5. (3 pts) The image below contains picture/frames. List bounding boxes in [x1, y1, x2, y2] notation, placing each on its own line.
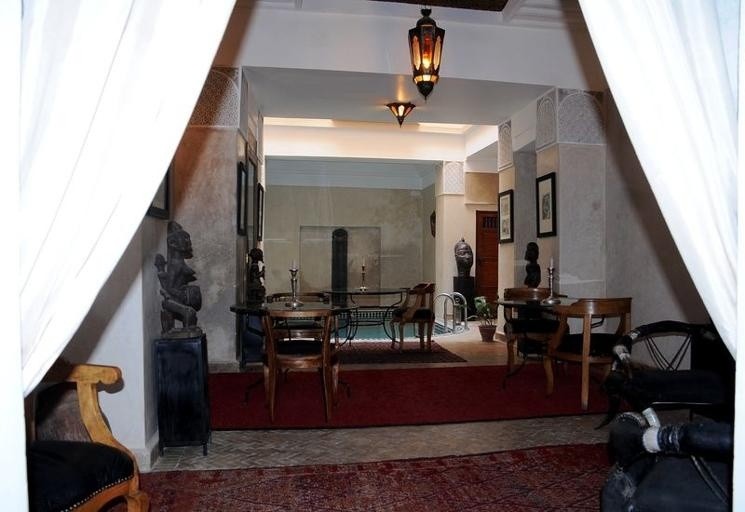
[149, 172, 172, 220]
[536, 172, 556, 236]
[257, 183, 263, 240]
[498, 189, 514, 242]
[238, 161, 246, 235]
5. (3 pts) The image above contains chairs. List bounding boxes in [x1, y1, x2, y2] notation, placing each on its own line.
[260, 284, 435, 423]
[612, 320, 731, 423]
[503, 288, 633, 410]
[24, 364, 150, 512]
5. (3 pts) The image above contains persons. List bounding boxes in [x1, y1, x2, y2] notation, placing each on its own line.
[454, 238, 474, 279]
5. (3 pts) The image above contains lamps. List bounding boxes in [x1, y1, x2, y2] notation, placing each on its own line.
[386, 105, 414, 128]
[407, 7, 445, 105]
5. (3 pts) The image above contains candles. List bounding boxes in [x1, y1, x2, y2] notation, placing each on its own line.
[549, 253, 554, 270]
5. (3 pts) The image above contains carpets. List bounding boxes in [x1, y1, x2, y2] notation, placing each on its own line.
[101, 442, 611, 512]
[327, 340, 468, 364]
[208, 362, 637, 431]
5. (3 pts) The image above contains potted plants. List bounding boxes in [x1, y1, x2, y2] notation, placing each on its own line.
[459, 300, 498, 341]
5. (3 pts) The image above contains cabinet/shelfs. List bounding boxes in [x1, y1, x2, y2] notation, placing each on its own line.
[239, 315, 267, 369]
